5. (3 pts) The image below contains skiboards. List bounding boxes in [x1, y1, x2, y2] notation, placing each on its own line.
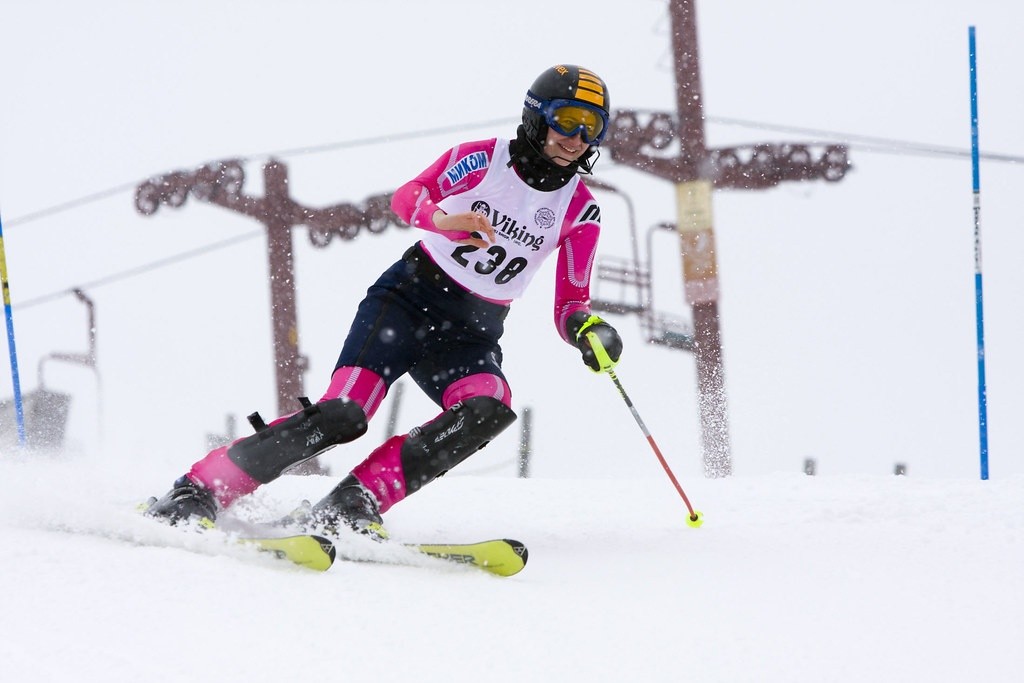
[26, 500, 529, 579]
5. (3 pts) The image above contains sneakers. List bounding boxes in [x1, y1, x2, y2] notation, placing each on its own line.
[313, 474, 390, 540]
[144, 475, 219, 532]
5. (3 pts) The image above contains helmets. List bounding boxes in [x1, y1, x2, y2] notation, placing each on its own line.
[523, 65, 610, 145]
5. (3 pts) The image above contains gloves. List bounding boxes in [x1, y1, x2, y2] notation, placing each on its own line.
[567, 313, 621, 371]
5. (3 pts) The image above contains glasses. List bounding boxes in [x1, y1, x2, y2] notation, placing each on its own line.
[525, 90, 609, 146]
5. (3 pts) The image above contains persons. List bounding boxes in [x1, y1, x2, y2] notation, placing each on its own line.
[151, 65, 622, 540]
